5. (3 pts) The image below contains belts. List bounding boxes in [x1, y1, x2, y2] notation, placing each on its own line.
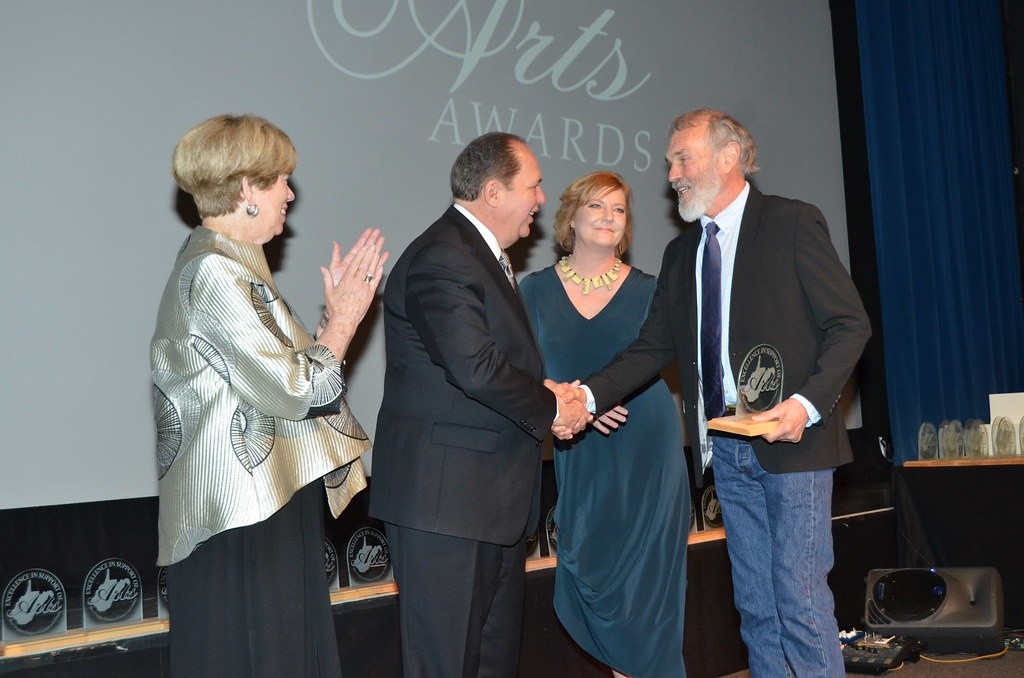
[723, 407, 736, 419]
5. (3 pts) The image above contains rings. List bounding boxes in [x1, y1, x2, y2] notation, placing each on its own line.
[365, 276, 373, 283]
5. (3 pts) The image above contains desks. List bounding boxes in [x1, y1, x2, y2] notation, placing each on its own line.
[893, 457, 1024, 633]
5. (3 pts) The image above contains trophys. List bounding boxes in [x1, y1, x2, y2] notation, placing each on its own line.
[707, 344, 784, 437]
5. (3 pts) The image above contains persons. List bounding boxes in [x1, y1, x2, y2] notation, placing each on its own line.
[368, 132, 591, 678]
[520, 172, 687, 678]
[559, 108, 874, 678]
[151, 114, 389, 678]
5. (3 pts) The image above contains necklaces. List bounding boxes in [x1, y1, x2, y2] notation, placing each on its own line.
[559, 253, 622, 295]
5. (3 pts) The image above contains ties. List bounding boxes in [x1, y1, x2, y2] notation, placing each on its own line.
[500, 249, 517, 296]
[701, 222, 725, 418]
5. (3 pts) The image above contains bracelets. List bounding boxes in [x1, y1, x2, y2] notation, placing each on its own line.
[320, 305, 329, 328]
